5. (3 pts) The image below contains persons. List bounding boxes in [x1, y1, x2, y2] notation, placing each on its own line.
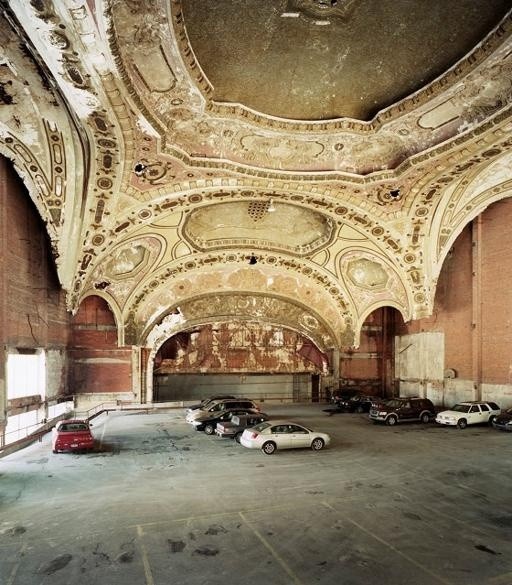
[325, 387, 331, 402]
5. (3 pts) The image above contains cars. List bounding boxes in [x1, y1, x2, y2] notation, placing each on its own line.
[239, 419, 330, 454]
[52, 420, 95, 453]
[331, 388, 384, 413]
[435, 401, 502, 430]
[186, 395, 270, 444]
[369, 397, 436, 425]
[492, 407, 512, 431]
